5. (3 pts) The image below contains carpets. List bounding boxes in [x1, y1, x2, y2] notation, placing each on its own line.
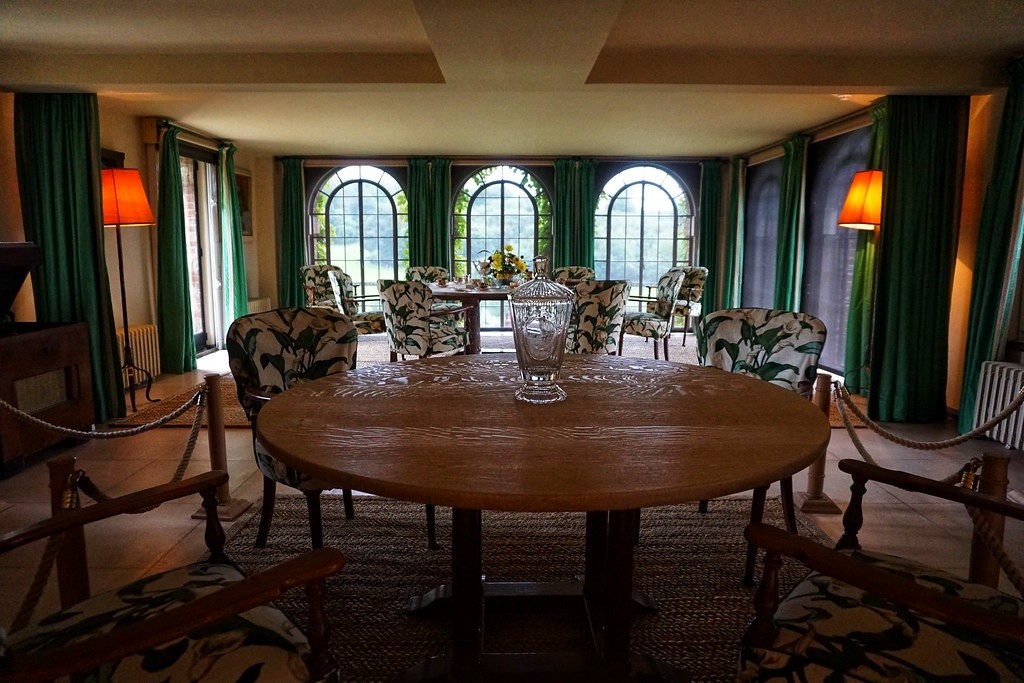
[812, 393, 868, 429]
[107, 369, 252, 429]
[195, 491, 837, 682]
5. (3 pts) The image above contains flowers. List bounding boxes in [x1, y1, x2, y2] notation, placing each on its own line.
[477, 245, 527, 284]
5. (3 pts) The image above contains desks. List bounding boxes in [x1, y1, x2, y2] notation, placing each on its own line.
[255, 353, 831, 682]
[427, 279, 514, 355]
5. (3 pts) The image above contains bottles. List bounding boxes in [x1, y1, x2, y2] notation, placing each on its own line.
[506, 252, 576, 407]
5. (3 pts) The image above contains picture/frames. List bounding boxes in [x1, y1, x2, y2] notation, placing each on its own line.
[233, 167, 255, 242]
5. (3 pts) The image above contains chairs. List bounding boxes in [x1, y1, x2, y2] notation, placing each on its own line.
[0, 468, 345, 683]
[696, 307, 827, 587]
[645, 267, 709, 347]
[619, 271, 686, 362]
[300, 264, 474, 362]
[737, 458, 1024, 683]
[226, 306, 441, 592]
[551, 266, 632, 356]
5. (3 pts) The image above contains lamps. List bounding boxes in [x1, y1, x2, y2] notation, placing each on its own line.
[100, 168, 161, 414]
[838, 170, 883, 396]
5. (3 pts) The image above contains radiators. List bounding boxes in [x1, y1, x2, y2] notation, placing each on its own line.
[115, 325, 161, 388]
[248, 297, 272, 314]
[971, 362, 1024, 450]
